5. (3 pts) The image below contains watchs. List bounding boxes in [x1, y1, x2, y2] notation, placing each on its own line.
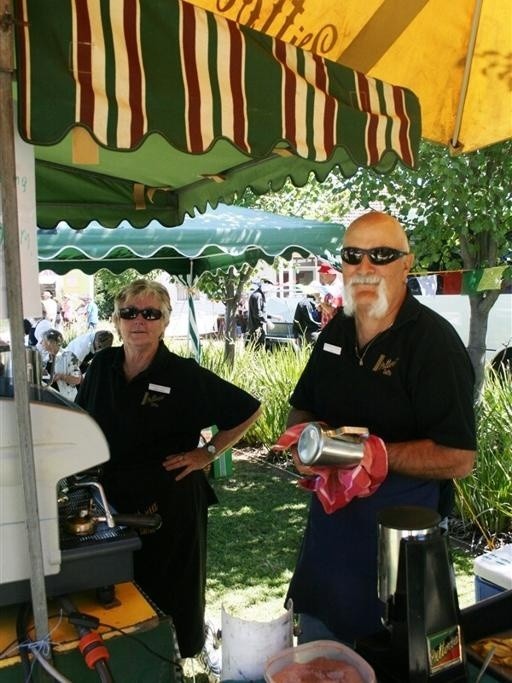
[202, 440, 220, 461]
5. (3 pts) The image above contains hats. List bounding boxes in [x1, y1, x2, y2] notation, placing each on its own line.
[317, 263, 338, 274]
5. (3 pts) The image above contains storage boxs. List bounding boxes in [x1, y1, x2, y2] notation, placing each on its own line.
[473, 542, 512, 603]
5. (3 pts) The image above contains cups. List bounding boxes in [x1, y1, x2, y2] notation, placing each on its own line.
[298, 422, 369, 471]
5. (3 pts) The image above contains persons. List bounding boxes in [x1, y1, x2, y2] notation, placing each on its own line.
[74, 277, 264, 668]
[235, 280, 342, 353]
[286, 210, 478, 652]
[1, 290, 116, 404]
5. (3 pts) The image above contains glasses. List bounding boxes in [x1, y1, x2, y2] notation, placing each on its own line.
[119, 307, 162, 320]
[341, 247, 408, 265]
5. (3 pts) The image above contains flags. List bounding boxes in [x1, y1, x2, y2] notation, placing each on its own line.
[411, 266, 509, 296]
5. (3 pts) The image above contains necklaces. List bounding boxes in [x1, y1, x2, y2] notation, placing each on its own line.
[354, 321, 392, 367]
